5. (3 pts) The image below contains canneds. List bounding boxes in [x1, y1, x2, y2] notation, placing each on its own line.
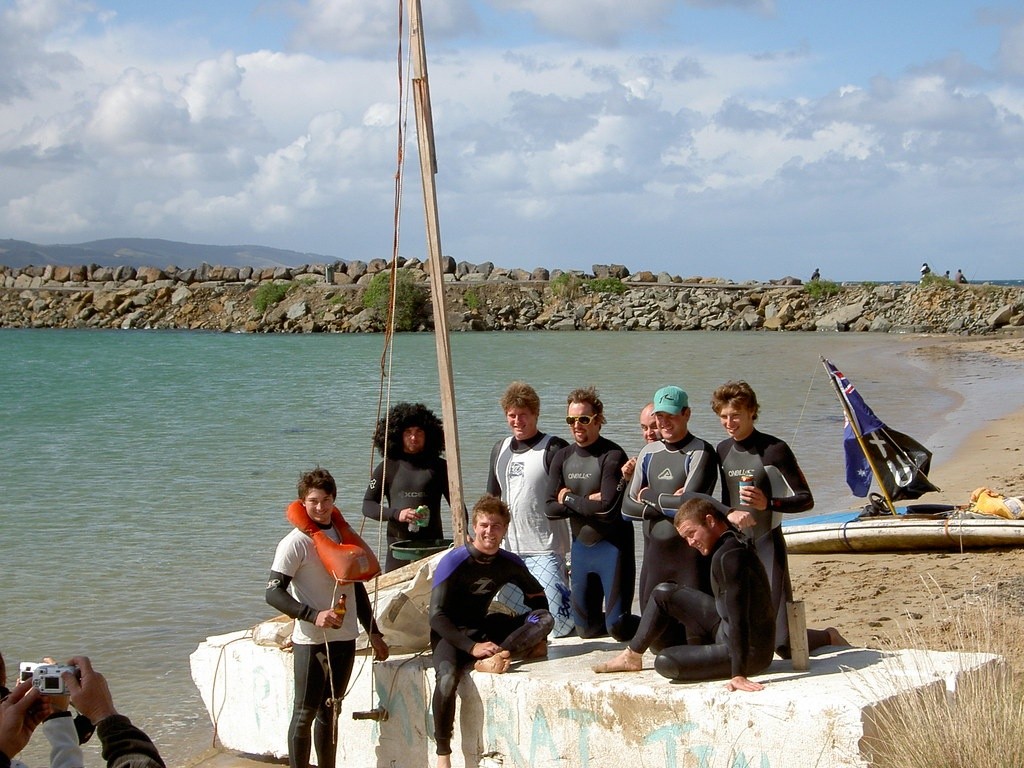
[739, 475, 755, 506]
[408, 505, 430, 532]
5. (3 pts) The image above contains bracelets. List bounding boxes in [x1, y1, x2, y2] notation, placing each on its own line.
[472, 643, 479, 652]
[90, 715, 110, 727]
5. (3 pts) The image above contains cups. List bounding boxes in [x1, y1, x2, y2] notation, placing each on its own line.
[739, 475, 754, 506]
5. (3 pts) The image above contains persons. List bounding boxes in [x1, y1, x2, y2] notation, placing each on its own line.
[0, 651, 166, 768]
[710, 380, 853, 660]
[590, 497, 774, 693]
[620, 386, 720, 656]
[921, 263, 932, 278]
[956, 269, 969, 284]
[363, 404, 474, 573]
[543, 385, 642, 644]
[941, 271, 950, 279]
[486, 381, 571, 638]
[811, 268, 820, 281]
[620, 402, 664, 614]
[428, 494, 554, 768]
[265, 472, 388, 768]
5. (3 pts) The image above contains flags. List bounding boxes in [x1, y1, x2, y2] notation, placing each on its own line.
[827, 359, 884, 501]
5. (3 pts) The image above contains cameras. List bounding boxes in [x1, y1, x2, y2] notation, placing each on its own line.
[19, 662, 37, 695]
[32, 665, 78, 695]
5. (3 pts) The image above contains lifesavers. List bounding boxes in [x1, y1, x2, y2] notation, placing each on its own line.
[286, 499, 382, 586]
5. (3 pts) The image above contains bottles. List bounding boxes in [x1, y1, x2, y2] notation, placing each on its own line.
[331, 594, 347, 629]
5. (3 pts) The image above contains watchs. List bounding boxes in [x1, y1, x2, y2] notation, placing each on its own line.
[766, 497, 774, 511]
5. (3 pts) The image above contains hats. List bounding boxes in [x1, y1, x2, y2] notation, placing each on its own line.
[651, 386, 688, 415]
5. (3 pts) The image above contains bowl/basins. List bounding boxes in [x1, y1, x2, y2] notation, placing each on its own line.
[390, 538, 454, 559]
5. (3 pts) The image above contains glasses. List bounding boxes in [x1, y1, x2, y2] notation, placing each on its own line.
[566, 413, 598, 425]
